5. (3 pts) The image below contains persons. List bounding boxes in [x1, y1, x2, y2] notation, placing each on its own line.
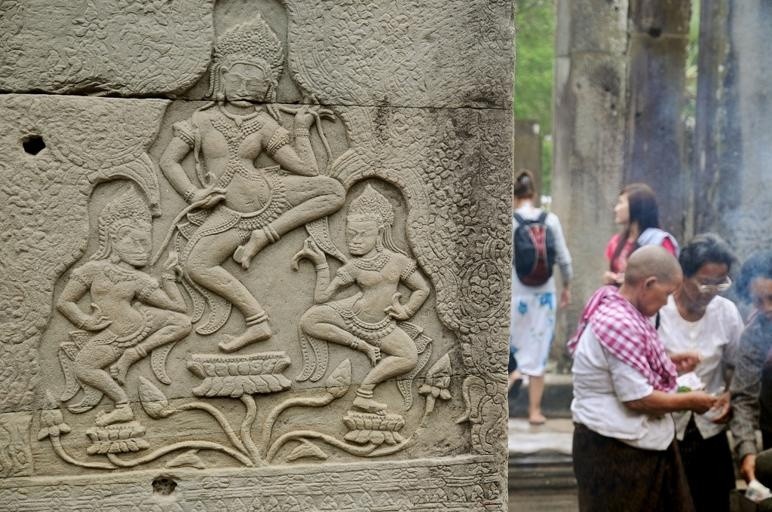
[52, 182, 191, 426]
[155, 11, 343, 353]
[286, 179, 431, 415]
[508, 166, 574, 427]
[723, 248, 770, 510]
[644, 230, 748, 510]
[569, 242, 720, 510]
[600, 181, 680, 290]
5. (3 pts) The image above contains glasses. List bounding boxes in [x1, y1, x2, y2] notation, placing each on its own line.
[690, 274, 733, 294]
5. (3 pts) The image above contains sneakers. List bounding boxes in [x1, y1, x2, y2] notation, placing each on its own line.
[528, 413, 547, 425]
[508, 373, 523, 400]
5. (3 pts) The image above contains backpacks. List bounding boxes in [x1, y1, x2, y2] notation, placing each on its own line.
[512, 210, 558, 288]
